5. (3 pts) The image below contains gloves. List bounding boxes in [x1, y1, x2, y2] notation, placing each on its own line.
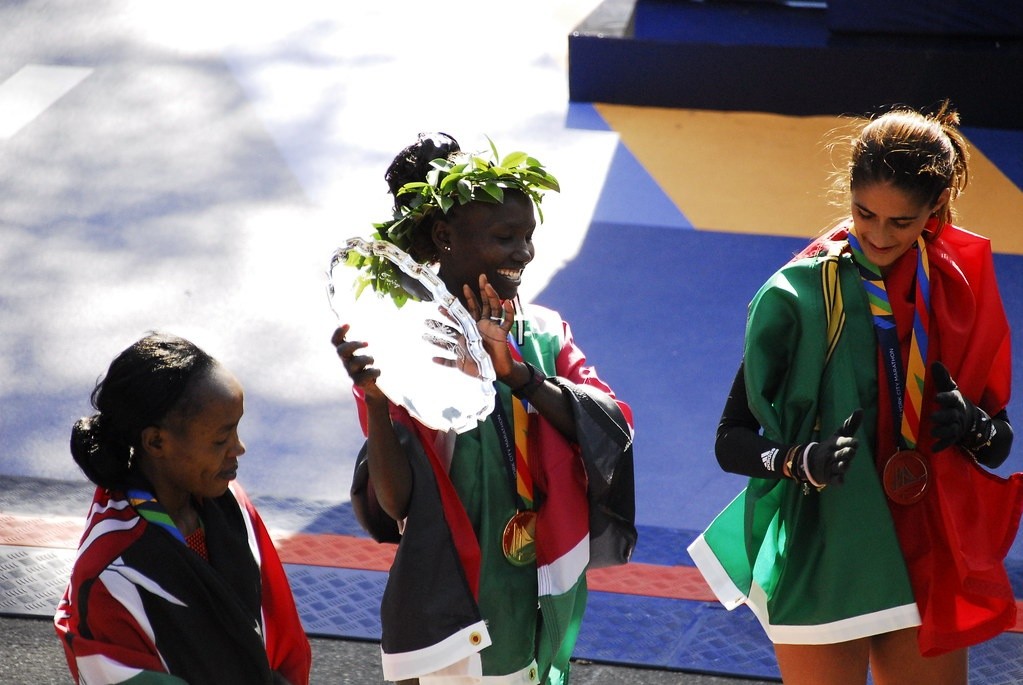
[807, 408, 863, 486]
[926, 363, 991, 455]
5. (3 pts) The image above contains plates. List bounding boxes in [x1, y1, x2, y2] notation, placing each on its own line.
[320, 233, 497, 434]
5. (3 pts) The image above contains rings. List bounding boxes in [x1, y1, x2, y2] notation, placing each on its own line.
[488, 315, 502, 321]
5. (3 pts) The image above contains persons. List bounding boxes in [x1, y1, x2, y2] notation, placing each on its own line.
[330, 129, 636, 685]
[715, 98, 1023, 685]
[53, 333, 311, 685]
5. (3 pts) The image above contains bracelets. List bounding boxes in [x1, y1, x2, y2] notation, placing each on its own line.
[957, 402, 994, 452]
[784, 440, 827, 494]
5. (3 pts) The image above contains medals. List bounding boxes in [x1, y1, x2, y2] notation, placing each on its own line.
[882, 449, 930, 506]
[501, 511, 540, 567]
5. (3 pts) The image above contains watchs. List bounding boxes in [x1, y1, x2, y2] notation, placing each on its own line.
[511, 361, 547, 402]
